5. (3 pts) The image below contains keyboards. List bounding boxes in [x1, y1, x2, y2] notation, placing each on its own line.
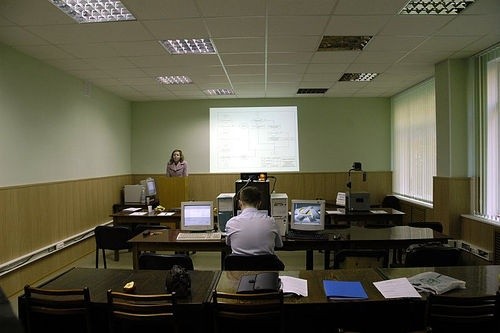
[176, 233, 221, 240]
[288, 232, 329, 241]
[333, 234, 338, 240]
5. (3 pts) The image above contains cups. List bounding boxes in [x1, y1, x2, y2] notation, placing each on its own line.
[148, 206, 153, 212]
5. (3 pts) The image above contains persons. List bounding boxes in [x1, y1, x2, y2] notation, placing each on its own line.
[165, 150, 188, 178]
[224, 185, 283, 257]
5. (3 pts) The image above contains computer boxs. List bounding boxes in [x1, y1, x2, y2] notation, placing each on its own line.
[216, 192, 236, 235]
[124, 184, 145, 202]
[270, 193, 288, 237]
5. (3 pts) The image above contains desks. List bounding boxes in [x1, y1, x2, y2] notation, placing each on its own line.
[18, 176, 500, 333]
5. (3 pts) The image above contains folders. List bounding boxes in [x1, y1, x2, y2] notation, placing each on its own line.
[322, 279, 368, 300]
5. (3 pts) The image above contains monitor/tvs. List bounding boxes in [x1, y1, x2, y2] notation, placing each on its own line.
[139, 179, 156, 196]
[181, 202, 214, 230]
[291, 199, 325, 231]
